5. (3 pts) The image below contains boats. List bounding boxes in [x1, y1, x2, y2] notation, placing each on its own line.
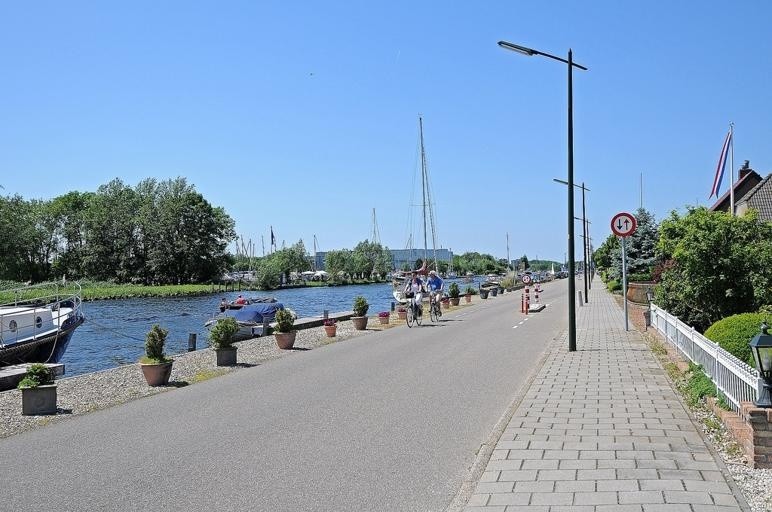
[202, 308, 298, 340]
[0, 274, 86, 365]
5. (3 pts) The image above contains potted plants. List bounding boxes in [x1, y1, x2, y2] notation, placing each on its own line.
[17, 362, 60, 415]
[480, 272, 524, 300]
[441, 280, 473, 311]
[270, 308, 298, 349]
[137, 323, 175, 386]
[325, 293, 405, 340]
[207, 315, 241, 367]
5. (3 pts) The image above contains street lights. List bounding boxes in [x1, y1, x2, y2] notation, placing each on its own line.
[495, 35, 596, 351]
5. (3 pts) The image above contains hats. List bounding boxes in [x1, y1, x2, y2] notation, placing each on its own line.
[428, 270, 436, 275]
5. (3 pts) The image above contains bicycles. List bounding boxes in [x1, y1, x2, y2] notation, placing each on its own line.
[429, 289, 440, 323]
[404, 291, 424, 328]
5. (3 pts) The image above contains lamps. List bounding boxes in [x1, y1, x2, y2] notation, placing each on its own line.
[745, 321, 772, 408]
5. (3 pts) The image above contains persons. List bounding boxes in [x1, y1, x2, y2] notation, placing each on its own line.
[221, 295, 245, 306]
[403, 272, 423, 322]
[425, 270, 444, 316]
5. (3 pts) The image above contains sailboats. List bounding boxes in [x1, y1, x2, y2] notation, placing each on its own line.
[391, 114, 444, 304]
